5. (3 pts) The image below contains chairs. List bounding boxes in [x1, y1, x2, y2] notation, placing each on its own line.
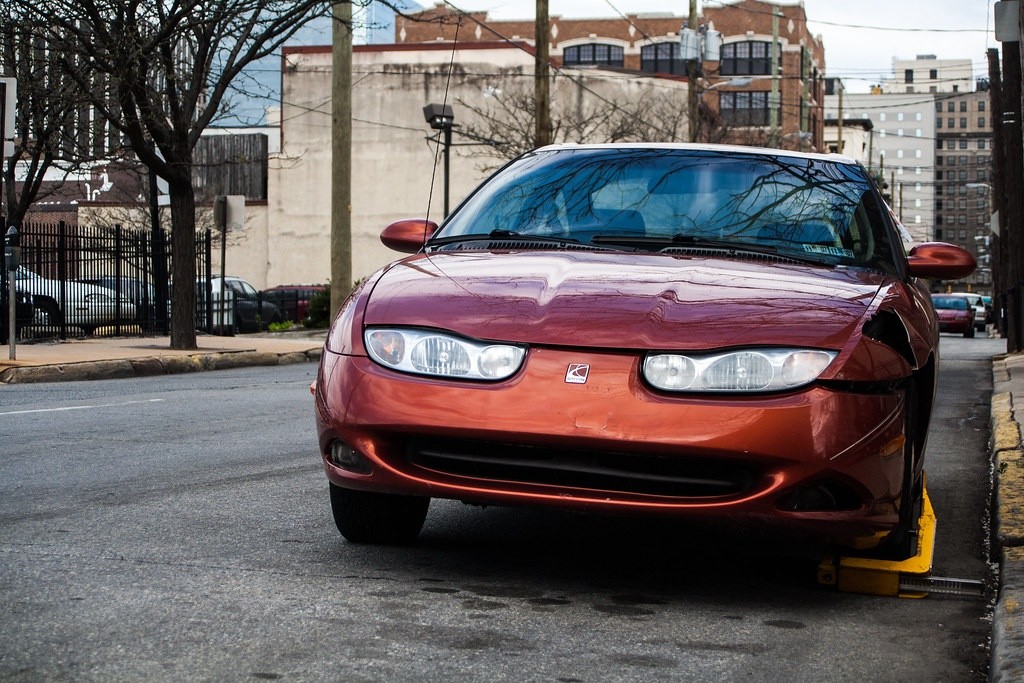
[756, 223, 834, 244]
[571, 208, 644, 232]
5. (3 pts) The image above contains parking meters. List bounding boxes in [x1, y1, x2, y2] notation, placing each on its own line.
[5, 225, 23, 358]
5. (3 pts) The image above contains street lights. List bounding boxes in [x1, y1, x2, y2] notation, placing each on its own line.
[771, 132, 807, 151]
[687, 79, 752, 143]
[424, 103, 453, 220]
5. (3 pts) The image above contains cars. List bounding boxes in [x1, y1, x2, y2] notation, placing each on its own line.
[956, 292, 991, 332]
[262, 285, 328, 319]
[982, 297, 994, 319]
[311, 139, 977, 584]
[1, 262, 137, 333]
[69, 275, 171, 322]
[929, 294, 977, 338]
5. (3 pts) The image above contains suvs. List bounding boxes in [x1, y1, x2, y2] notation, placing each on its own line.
[165, 275, 283, 329]
